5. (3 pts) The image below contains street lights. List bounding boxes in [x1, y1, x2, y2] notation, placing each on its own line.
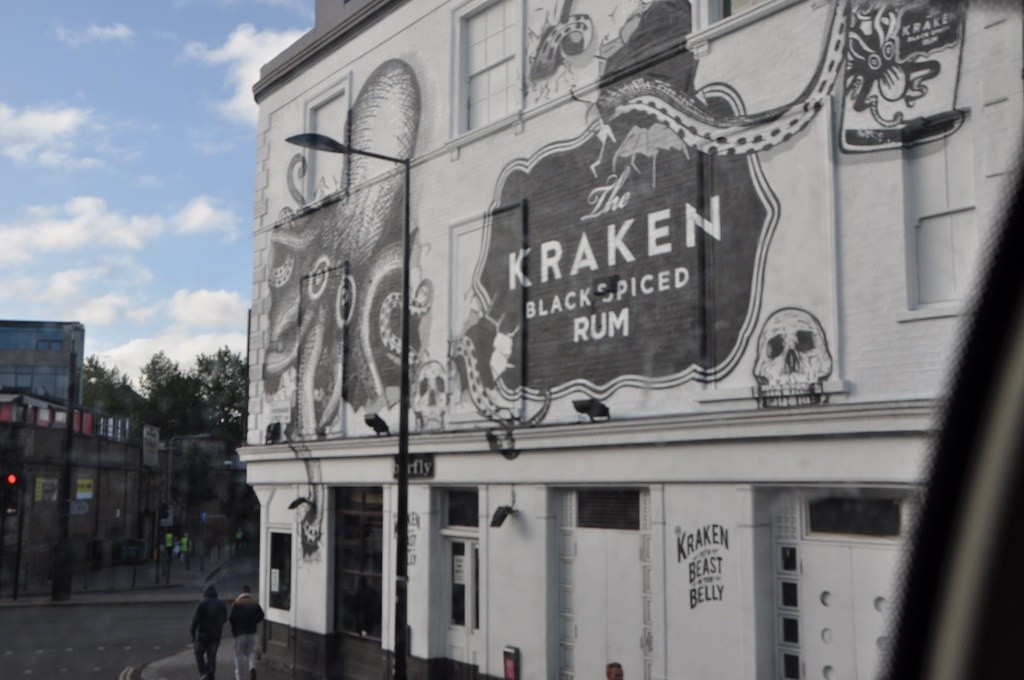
[160, 432, 212, 577]
[285, 130, 411, 679]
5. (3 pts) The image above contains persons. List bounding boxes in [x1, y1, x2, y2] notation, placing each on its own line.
[180, 532, 193, 565]
[164, 529, 174, 563]
[235, 527, 244, 552]
[190, 583, 228, 680]
[229, 585, 264, 680]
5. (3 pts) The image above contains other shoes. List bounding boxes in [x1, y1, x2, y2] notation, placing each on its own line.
[201, 673, 209, 680]
[251, 668, 258, 680]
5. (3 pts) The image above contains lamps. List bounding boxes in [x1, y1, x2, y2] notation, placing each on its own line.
[365, 413, 390, 436]
[265, 422, 280, 445]
[490, 506, 515, 527]
[572, 399, 611, 423]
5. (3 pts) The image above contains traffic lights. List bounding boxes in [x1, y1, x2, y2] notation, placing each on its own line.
[7, 463, 20, 496]
